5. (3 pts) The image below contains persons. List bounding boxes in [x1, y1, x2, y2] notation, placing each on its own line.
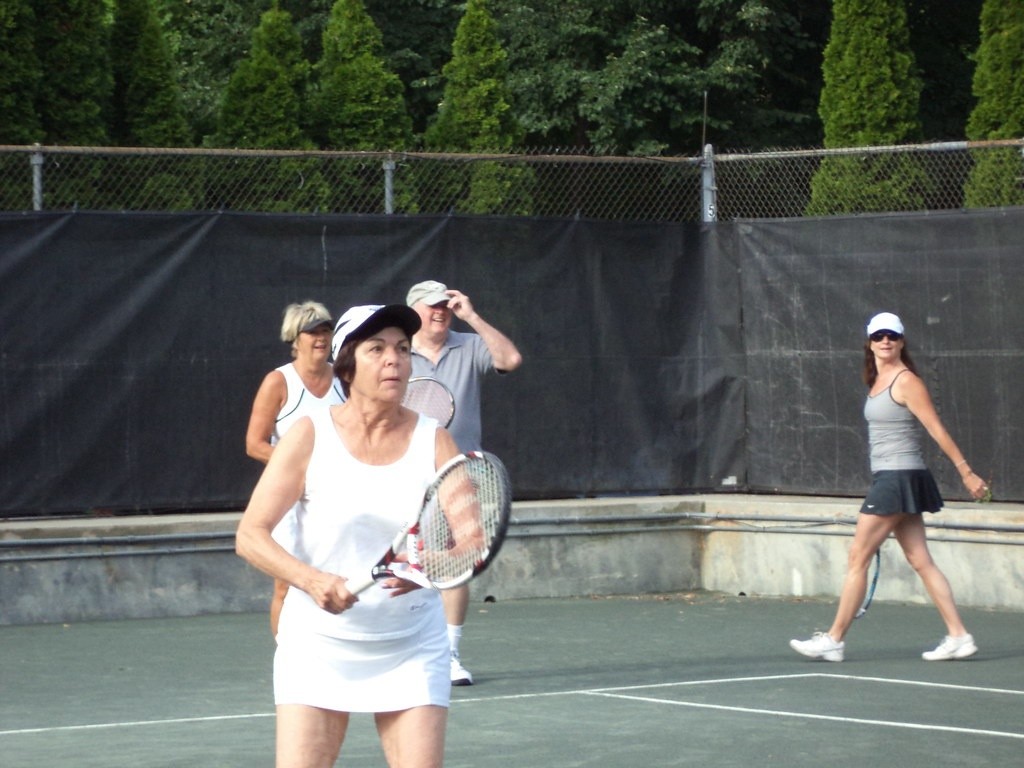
[234, 302, 488, 768]
[789, 311, 991, 663]
[402, 279, 523, 686]
[244, 298, 348, 647]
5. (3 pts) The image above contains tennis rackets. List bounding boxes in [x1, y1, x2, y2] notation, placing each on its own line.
[343, 450, 513, 598]
[400, 376, 458, 430]
[852, 548, 881, 618]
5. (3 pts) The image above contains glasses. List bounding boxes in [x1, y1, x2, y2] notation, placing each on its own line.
[871, 332, 899, 342]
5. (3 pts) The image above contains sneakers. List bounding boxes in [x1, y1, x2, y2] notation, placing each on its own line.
[449, 660, 473, 686]
[922, 633, 978, 661]
[790, 632, 845, 662]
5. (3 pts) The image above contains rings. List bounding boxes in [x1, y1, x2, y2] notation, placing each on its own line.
[982, 486, 988, 491]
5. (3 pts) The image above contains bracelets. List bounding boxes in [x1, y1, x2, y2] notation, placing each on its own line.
[955, 459, 967, 468]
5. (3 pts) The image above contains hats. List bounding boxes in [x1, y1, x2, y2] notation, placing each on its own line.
[283, 317, 338, 345]
[406, 280, 454, 307]
[331, 304, 423, 364]
[867, 312, 905, 339]
